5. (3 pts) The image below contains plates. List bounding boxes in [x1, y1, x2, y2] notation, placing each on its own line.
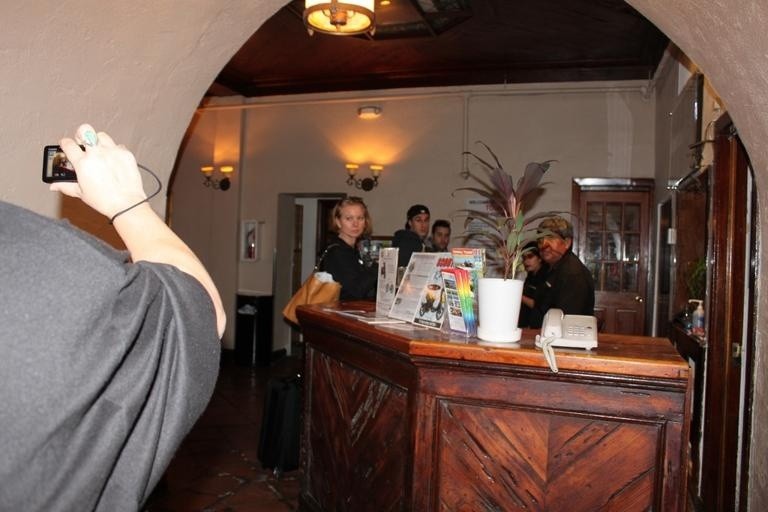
[476, 326, 523, 343]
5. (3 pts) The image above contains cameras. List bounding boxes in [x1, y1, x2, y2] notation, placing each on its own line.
[43, 145, 85, 183]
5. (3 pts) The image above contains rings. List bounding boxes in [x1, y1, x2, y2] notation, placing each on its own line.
[80, 125, 101, 146]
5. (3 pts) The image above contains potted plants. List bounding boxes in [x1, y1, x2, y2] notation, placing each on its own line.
[681, 256, 708, 326]
[451, 138, 560, 341]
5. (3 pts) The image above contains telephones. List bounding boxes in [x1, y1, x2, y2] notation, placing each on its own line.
[534, 308, 598, 373]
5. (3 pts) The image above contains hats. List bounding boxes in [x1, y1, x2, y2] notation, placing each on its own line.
[404, 202, 433, 232]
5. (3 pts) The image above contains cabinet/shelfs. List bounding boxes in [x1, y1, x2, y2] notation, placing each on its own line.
[570, 177, 657, 338]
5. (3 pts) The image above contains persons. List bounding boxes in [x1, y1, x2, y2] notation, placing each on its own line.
[317, 195, 378, 300]
[535, 216, 596, 328]
[390, 203, 432, 270]
[0, 124, 229, 512]
[516, 240, 558, 330]
[429, 219, 452, 253]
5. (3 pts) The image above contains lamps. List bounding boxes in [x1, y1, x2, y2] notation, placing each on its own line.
[369, 163, 384, 189]
[219, 164, 234, 193]
[306, 0, 379, 37]
[198, 164, 217, 191]
[345, 163, 364, 189]
